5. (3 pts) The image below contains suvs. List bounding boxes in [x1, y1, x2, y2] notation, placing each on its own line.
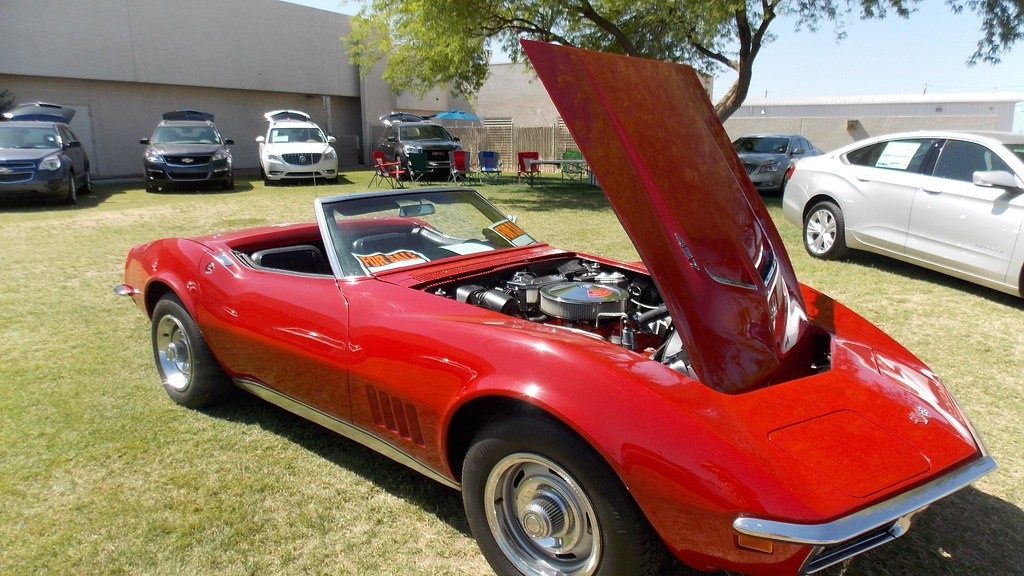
[374, 111, 466, 185]
[256, 110, 340, 186]
[0, 102, 94, 205]
[139, 111, 234, 194]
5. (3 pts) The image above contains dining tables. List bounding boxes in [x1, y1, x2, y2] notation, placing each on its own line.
[446, 150, 477, 186]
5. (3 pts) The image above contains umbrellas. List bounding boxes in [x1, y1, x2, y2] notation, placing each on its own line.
[428, 110, 481, 126]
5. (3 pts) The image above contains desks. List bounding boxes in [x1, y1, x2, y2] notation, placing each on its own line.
[530, 160, 596, 189]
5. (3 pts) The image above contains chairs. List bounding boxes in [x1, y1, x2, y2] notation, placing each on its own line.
[368, 150, 405, 189]
[561, 151, 586, 185]
[352, 231, 413, 273]
[517, 152, 541, 185]
[298, 130, 322, 141]
[199, 131, 211, 139]
[476, 151, 504, 186]
[250, 244, 329, 275]
[405, 153, 439, 187]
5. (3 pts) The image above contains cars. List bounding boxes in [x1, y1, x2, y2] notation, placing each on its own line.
[728, 131, 825, 195]
[110, 37, 996, 576]
[783, 130, 1023, 304]
